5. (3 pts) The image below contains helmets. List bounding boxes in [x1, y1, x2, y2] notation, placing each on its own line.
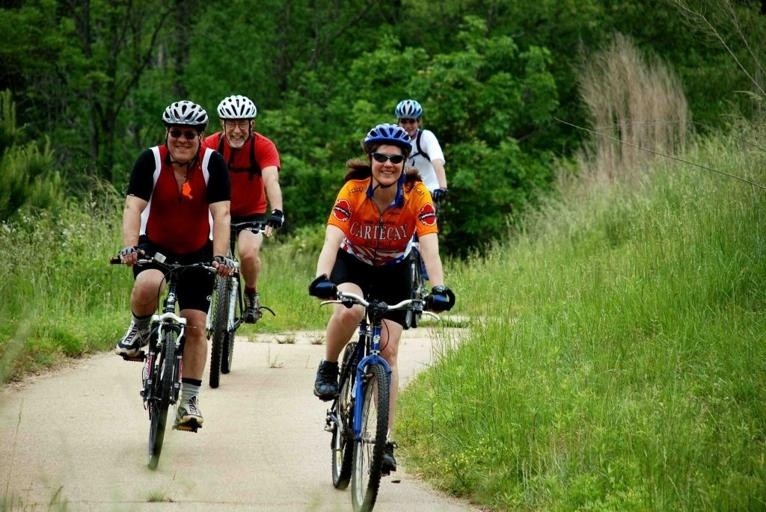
[162, 100, 209, 128]
[395, 99, 422, 121]
[217, 95, 257, 119]
[364, 123, 413, 147]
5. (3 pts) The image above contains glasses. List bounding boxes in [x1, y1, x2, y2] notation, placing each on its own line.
[171, 130, 195, 139]
[371, 152, 403, 164]
[401, 118, 415, 124]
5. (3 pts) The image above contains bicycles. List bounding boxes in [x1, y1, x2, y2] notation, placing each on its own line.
[206, 222, 262, 388]
[398, 188, 450, 331]
[109, 248, 239, 470]
[318, 291, 441, 511]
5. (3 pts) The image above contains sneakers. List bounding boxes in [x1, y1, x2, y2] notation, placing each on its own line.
[383, 442, 399, 471]
[175, 396, 204, 428]
[315, 361, 341, 395]
[114, 313, 151, 358]
[243, 293, 259, 324]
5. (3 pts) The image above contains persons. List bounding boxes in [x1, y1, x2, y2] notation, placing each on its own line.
[200, 93, 285, 324]
[313, 120, 457, 477]
[113, 97, 235, 433]
[392, 99, 448, 281]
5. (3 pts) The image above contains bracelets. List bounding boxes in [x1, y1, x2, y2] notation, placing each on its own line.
[271, 209, 281, 215]
